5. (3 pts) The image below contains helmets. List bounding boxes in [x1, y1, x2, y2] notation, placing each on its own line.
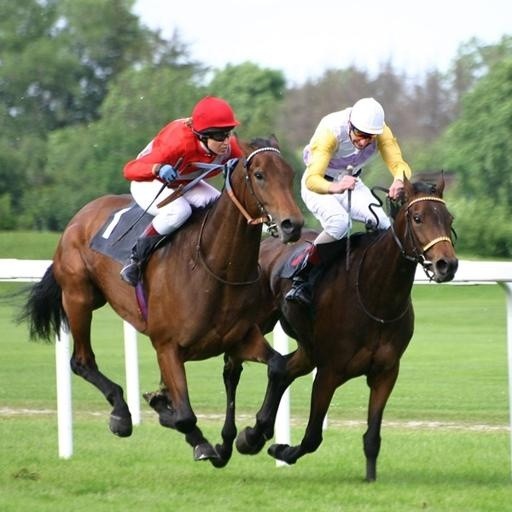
[350, 97, 384, 134]
[192, 96, 239, 132]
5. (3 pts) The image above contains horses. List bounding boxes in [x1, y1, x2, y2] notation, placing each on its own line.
[1, 131, 306, 462]
[211, 166, 462, 483]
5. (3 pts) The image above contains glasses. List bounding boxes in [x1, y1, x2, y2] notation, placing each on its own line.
[353, 127, 377, 138]
[205, 130, 232, 141]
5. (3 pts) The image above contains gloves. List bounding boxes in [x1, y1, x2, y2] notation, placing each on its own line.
[155, 164, 179, 183]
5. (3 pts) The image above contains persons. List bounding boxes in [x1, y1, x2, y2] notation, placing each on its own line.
[119, 96, 246, 287]
[285, 98, 412, 305]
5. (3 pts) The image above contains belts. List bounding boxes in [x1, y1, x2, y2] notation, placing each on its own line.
[325, 168, 361, 181]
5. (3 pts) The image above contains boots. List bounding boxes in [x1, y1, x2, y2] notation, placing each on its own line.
[285, 244, 320, 303]
[119, 223, 163, 286]
